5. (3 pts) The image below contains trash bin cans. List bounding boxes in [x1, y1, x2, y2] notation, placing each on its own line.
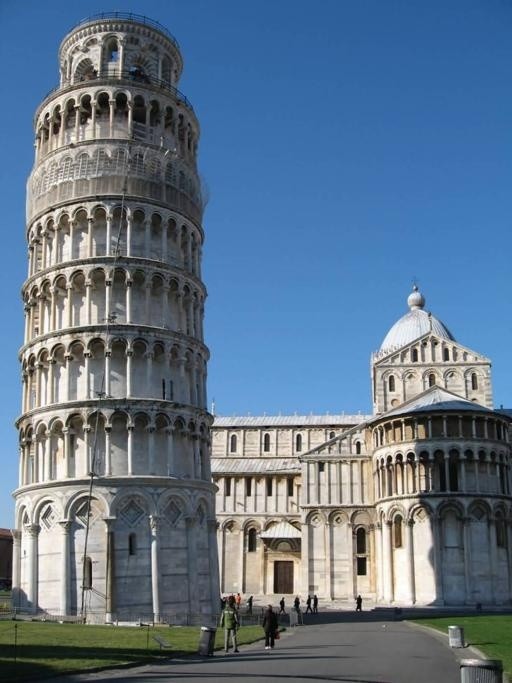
[448, 625, 464, 648]
[459, 658, 504, 683]
[198, 626, 217, 657]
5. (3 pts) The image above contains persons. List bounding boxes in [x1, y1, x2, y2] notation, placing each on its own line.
[262, 605, 278, 650]
[219, 601, 240, 653]
[222, 593, 253, 615]
[305, 595, 312, 614]
[279, 597, 286, 613]
[356, 595, 362, 611]
[313, 594, 318, 614]
[294, 598, 300, 612]
